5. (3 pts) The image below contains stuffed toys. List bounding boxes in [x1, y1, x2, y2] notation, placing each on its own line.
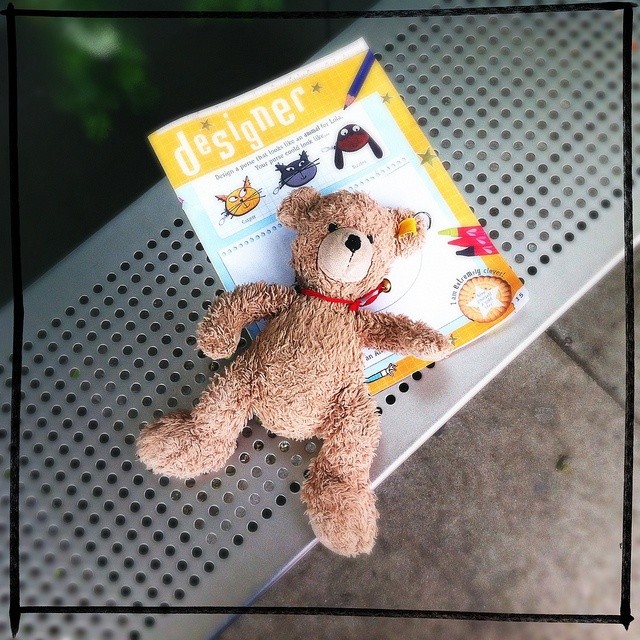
[135, 184, 453, 558]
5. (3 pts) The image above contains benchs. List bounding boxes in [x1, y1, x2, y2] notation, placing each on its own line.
[1, 1, 640, 640]
